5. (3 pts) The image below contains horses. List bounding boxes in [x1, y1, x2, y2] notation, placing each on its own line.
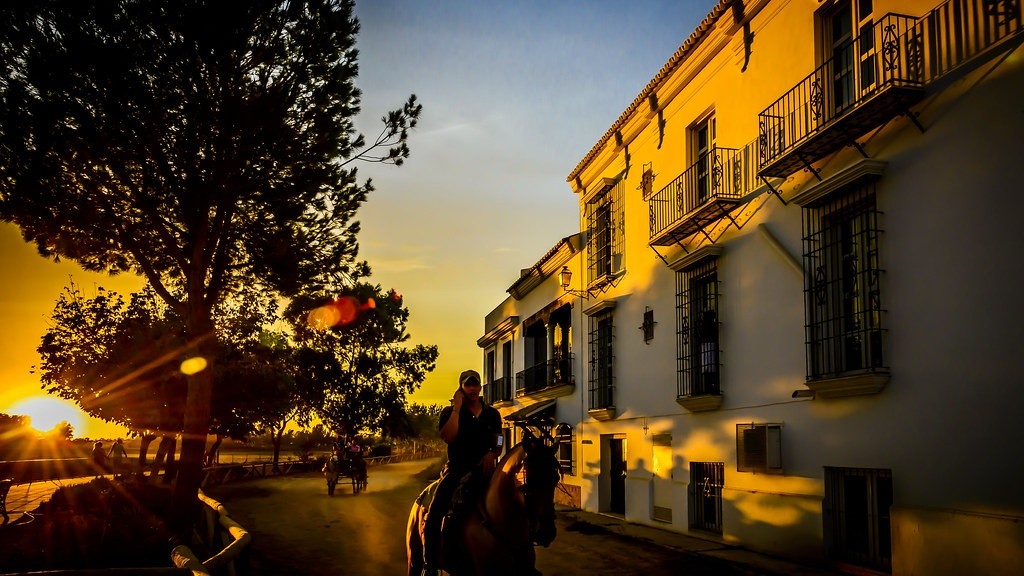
[405, 434, 562, 576]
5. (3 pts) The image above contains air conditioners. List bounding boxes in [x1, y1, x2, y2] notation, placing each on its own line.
[743, 429, 781, 469]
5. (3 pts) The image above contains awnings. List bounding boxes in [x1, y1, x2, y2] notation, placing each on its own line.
[503, 398, 558, 424]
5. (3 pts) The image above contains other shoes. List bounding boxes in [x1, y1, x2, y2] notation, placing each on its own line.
[421, 568, 438, 576]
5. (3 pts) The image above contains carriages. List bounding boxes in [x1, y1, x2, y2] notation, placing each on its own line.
[321, 441, 370, 496]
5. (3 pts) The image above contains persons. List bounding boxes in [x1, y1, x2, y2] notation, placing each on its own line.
[337, 435, 361, 457]
[419, 371, 502, 576]
[88, 438, 128, 479]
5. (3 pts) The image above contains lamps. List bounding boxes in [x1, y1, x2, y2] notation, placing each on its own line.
[558, 265, 590, 303]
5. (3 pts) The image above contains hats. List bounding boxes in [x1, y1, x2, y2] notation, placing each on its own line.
[460, 370, 480, 383]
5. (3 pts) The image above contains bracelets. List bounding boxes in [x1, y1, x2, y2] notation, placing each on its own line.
[453, 408, 460, 412]
[489, 448, 497, 453]
